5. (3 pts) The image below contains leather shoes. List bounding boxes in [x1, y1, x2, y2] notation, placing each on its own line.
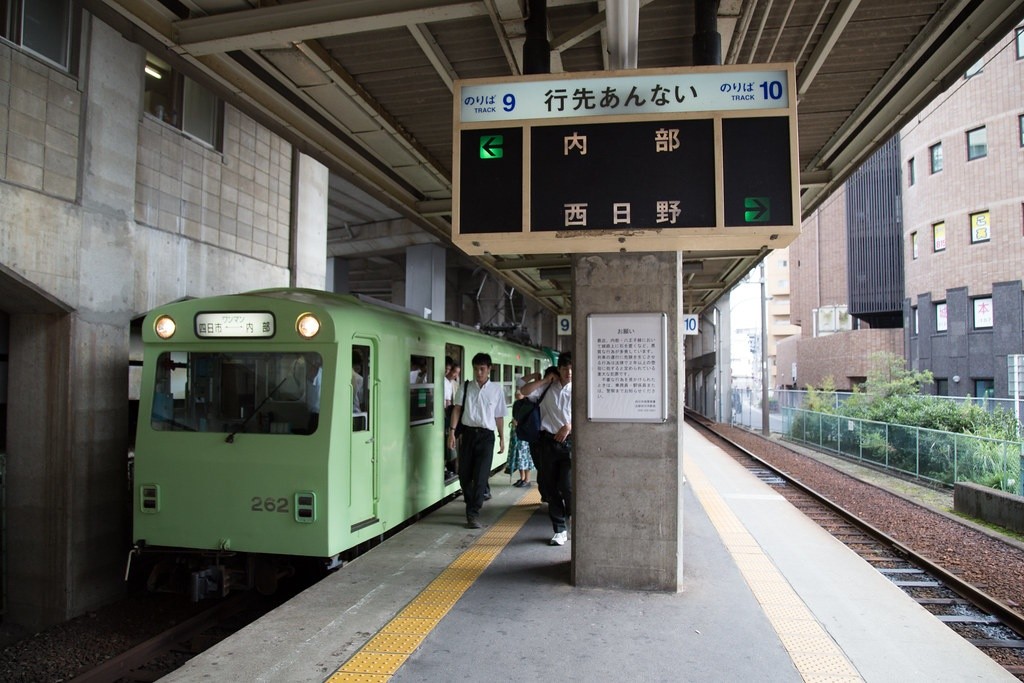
[514, 480, 531, 488]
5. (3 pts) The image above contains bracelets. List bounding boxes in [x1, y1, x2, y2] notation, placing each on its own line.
[449, 427, 456, 431]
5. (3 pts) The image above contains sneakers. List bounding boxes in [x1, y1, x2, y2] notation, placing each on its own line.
[467, 515, 481, 529]
[551, 530, 567, 546]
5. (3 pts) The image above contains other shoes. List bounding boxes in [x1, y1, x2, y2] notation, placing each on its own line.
[482, 491, 493, 500]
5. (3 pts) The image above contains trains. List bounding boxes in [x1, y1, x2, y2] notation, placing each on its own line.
[124, 285, 563, 623]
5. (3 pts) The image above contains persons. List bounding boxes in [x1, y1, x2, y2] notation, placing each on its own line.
[521, 351, 573, 546]
[446, 360, 460, 405]
[504, 390, 536, 487]
[516, 365, 558, 397]
[444, 356, 452, 408]
[352, 367, 364, 412]
[448, 353, 508, 527]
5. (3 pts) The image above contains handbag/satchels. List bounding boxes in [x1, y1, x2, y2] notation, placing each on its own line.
[512, 399, 541, 437]
